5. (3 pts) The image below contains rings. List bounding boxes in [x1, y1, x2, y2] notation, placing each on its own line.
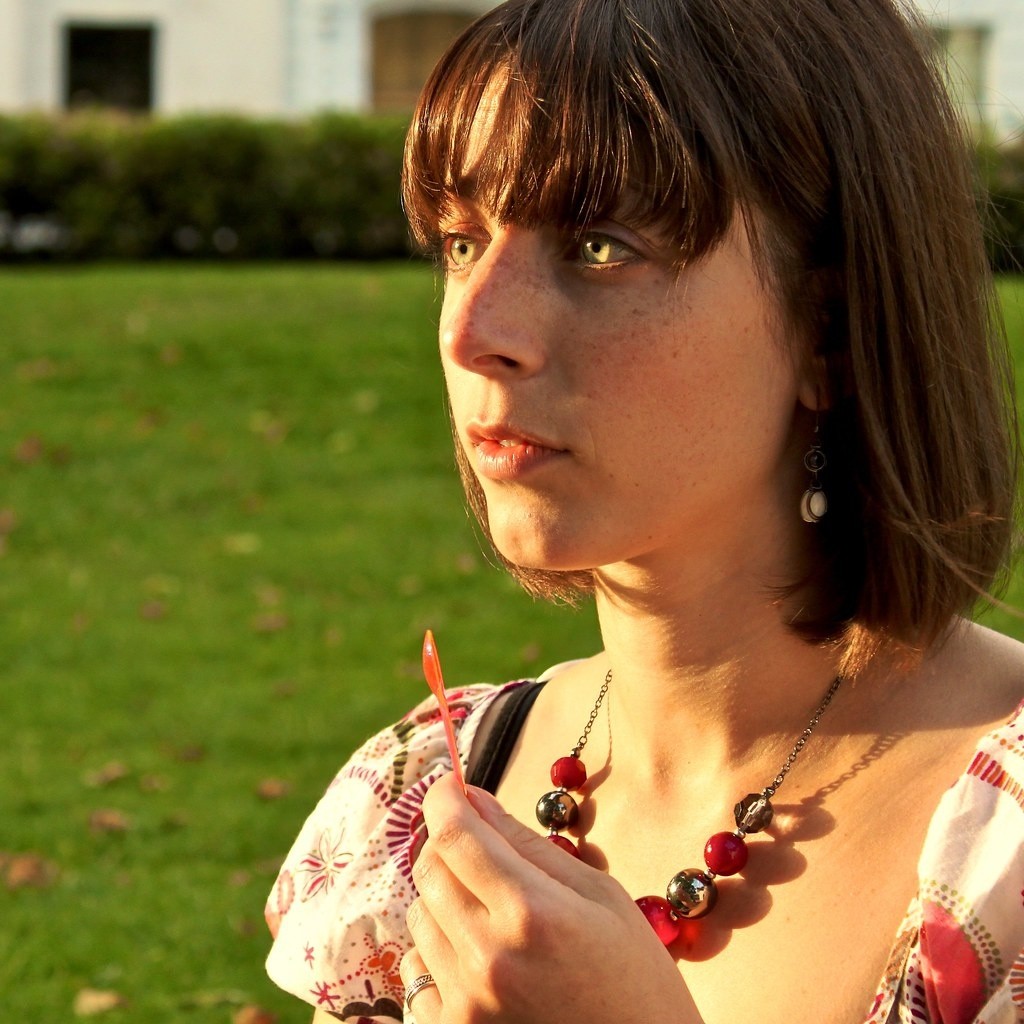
[404, 973, 436, 1011]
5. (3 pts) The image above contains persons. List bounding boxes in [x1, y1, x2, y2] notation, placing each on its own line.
[264, 0, 1024, 1024]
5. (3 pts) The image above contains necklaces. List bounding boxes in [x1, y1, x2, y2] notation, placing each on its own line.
[535, 651, 847, 946]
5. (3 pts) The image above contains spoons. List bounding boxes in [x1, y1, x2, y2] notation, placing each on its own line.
[422, 629, 468, 798]
